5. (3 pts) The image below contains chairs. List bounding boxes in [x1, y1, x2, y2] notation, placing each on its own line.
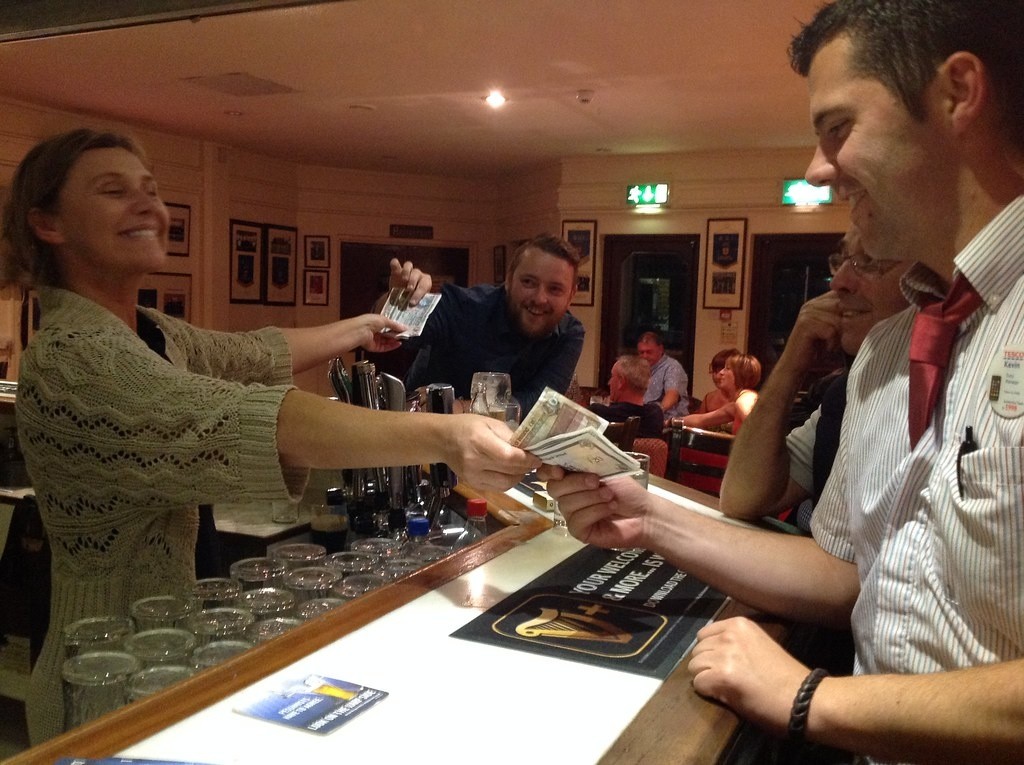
[603, 416, 735, 498]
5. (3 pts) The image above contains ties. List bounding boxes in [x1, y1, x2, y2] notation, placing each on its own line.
[906, 269, 985, 453]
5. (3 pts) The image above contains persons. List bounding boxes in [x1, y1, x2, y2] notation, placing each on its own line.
[699, 348, 739, 433]
[372, 232, 586, 425]
[664, 355, 761, 435]
[587, 356, 664, 438]
[720, 225, 916, 528]
[535, 0, 1024, 765]
[637, 333, 688, 417]
[0, 128, 543, 747]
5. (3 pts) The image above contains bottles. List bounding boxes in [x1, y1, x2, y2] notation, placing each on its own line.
[469, 387, 490, 423]
[400, 516, 430, 551]
[451, 499, 488, 554]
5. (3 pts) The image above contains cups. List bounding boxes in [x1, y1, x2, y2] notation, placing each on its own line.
[553, 500, 568, 533]
[487, 402, 521, 436]
[621, 452, 650, 492]
[590, 396, 603, 406]
[309, 503, 349, 555]
[63, 538, 454, 735]
[470, 371, 511, 422]
[672, 418, 683, 433]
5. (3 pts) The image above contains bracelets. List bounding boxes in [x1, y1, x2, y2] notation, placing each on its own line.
[789, 669, 829, 737]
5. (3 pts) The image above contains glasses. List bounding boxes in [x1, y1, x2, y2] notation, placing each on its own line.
[828, 251, 901, 279]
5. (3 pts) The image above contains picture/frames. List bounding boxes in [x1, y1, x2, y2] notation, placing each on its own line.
[703, 217, 747, 310]
[304, 234, 331, 268]
[493, 244, 505, 283]
[303, 269, 329, 306]
[163, 202, 191, 257]
[229, 219, 264, 304]
[264, 223, 297, 306]
[562, 219, 597, 307]
